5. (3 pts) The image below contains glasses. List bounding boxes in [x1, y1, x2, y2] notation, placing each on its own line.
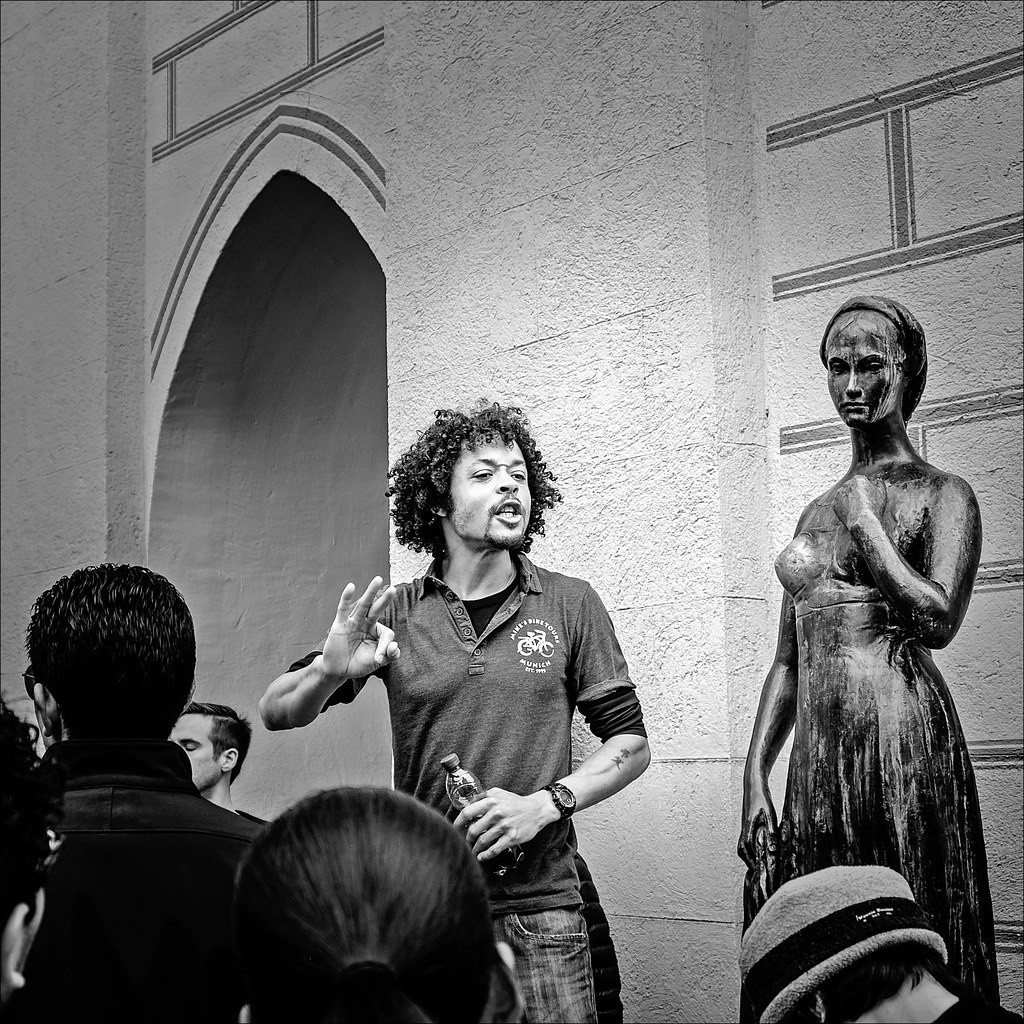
[21, 662, 60, 711]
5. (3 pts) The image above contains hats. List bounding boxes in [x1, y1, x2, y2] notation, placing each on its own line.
[738, 866, 950, 1024]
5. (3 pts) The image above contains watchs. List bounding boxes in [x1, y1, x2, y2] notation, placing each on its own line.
[539, 782, 577, 824]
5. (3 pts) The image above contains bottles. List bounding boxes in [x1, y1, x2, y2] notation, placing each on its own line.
[440, 753, 524, 878]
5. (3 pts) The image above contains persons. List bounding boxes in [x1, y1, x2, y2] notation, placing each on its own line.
[0, 695, 68, 1022]
[167, 702, 271, 827]
[737, 867, 1024, 1023]
[232, 785, 526, 1024]
[258, 400, 652, 1024]
[0, 563, 268, 1024]
[736, 294, 1001, 1006]
